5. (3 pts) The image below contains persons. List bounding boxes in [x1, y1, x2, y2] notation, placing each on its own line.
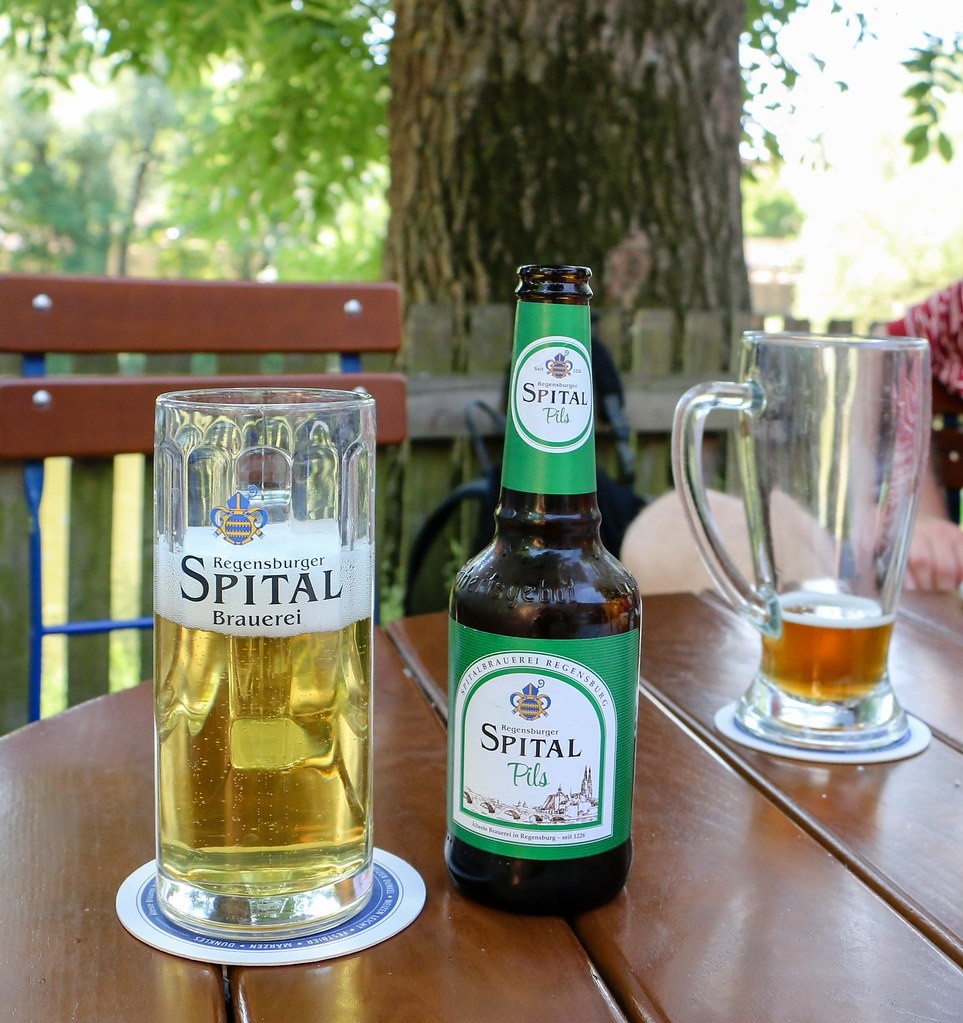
[620, 281, 960, 594]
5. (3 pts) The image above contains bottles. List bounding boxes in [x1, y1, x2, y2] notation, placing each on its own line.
[437, 259, 649, 919]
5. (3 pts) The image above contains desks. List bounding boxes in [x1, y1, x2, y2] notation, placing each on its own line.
[0, 581, 963, 1023]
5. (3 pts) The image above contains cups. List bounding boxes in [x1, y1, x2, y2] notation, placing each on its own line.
[673, 324, 931, 750]
[145, 385, 382, 941]
